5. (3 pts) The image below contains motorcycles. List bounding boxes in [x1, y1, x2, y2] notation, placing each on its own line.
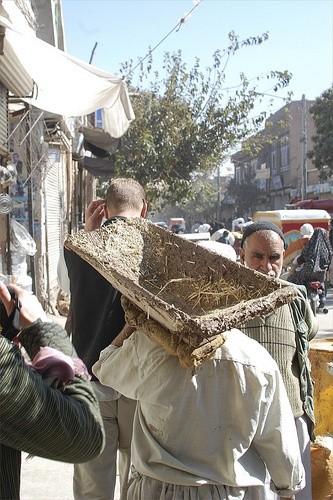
[252, 210, 332, 240]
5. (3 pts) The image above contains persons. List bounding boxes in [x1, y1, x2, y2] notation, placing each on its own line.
[0, 280, 106, 500]
[64, 178, 333, 500]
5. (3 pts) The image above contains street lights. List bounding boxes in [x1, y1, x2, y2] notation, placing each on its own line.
[249, 89, 308, 201]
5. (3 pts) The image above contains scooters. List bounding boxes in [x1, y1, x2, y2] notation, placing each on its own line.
[307, 281, 327, 316]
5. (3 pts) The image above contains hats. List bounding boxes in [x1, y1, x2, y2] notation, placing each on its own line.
[300, 223, 314, 240]
[240, 221, 288, 251]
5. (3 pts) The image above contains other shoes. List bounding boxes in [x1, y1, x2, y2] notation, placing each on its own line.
[319, 300, 326, 308]
